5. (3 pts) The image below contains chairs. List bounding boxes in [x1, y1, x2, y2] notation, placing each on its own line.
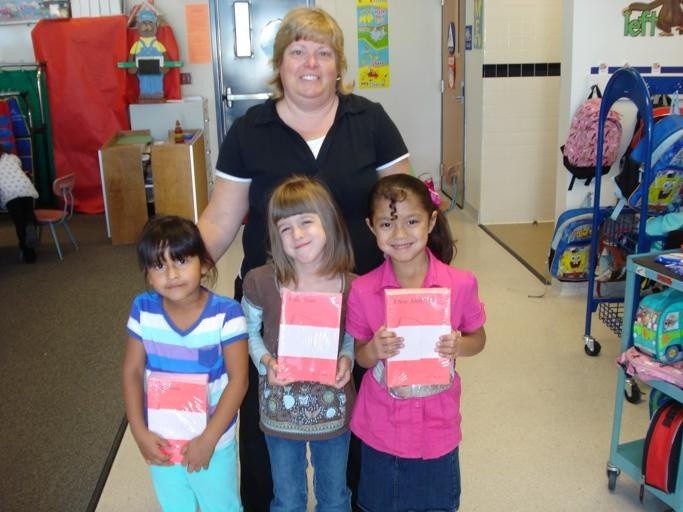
[19, 172, 80, 262]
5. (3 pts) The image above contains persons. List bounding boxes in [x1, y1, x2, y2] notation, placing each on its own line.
[345, 174, 487, 512]
[121, 215, 252, 512]
[238, 178, 360, 512]
[0, 143, 40, 264]
[196, 7, 409, 511]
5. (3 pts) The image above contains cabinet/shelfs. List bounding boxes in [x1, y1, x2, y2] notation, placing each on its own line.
[605, 248, 681, 512]
[129, 96, 214, 200]
[150, 127, 208, 225]
[579, 66, 681, 357]
[97, 129, 152, 246]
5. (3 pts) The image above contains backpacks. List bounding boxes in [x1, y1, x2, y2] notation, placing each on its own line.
[548, 193, 612, 283]
[621, 93, 683, 168]
[560, 85, 622, 179]
[610, 91, 683, 216]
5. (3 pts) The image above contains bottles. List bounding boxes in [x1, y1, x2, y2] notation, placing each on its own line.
[174, 120, 184, 142]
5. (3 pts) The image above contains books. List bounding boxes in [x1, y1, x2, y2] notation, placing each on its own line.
[382, 286, 453, 390]
[276, 288, 342, 385]
[146, 371, 209, 466]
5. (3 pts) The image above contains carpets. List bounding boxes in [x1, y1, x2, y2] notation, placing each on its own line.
[0, 204, 149, 510]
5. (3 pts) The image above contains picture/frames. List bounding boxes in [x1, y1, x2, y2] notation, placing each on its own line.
[0, 0, 71, 26]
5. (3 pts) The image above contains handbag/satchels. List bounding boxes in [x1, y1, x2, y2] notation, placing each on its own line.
[595, 215, 640, 283]
[633, 286, 683, 364]
[640, 399, 682, 494]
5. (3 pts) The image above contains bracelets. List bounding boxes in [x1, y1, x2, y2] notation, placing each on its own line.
[266, 357, 273, 368]
[200, 433, 215, 451]
